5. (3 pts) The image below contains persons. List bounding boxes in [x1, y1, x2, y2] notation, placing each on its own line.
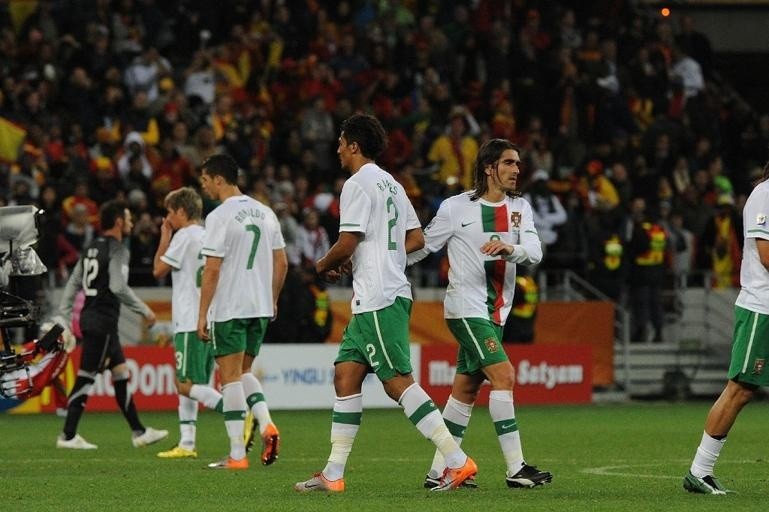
[406, 138, 552, 487]
[681, 161, 768, 495]
[151, 185, 257, 458]
[293, 110, 478, 491]
[56, 199, 170, 451]
[197, 151, 289, 470]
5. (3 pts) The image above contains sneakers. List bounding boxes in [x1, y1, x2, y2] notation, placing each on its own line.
[682, 469, 739, 495]
[131, 427, 169, 449]
[294, 470, 347, 495]
[207, 454, 251, 470]
[56, 432, 99, 451]
[504, 463, 553, 490]
[243, 412, 258, 454]
[156, 445, 198, 459]
[425, 456, 479, 494]
[260, 420, 280, 467]
